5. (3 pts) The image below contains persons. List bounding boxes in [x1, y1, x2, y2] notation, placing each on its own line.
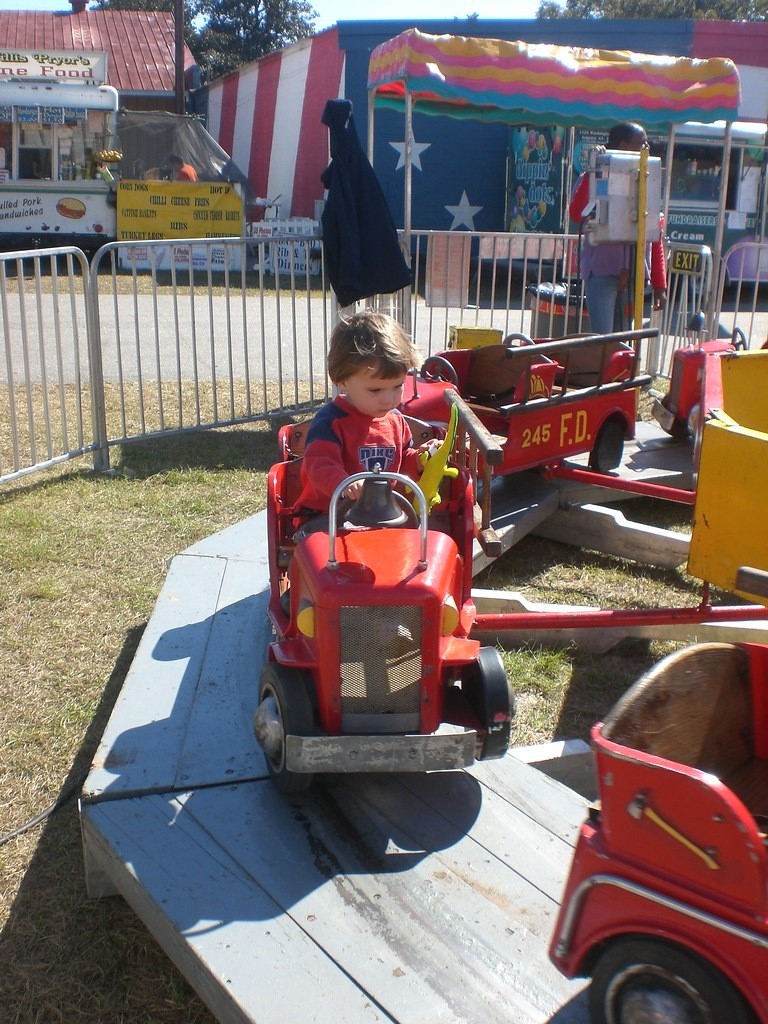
[569, 121, 668, 343]
[167, 154, 198, 181]
[279, 312, 445, 617]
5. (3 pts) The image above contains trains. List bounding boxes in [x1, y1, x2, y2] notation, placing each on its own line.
[250, 420, 519, 790]
[395, 328, 666, 479]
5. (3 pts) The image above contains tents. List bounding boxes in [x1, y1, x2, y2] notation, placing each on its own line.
[366, 27, 741, 340]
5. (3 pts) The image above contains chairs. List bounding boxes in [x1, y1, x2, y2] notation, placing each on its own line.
[267, 413, 475, 568]
[521, 333, 636, 390]
[421, 345, 558, 419]
[591, 640, 767, 920]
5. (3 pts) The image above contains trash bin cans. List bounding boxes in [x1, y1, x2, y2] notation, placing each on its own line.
[525, 282, 639, 344]
